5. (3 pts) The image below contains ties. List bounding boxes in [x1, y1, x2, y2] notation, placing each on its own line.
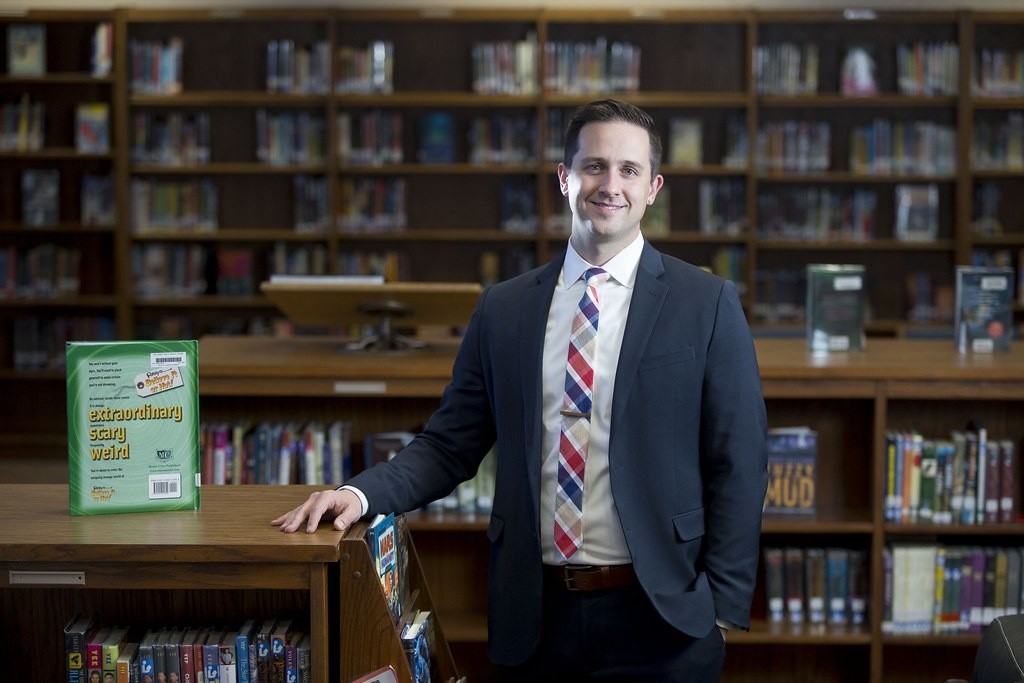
[554, 268, 612, 561]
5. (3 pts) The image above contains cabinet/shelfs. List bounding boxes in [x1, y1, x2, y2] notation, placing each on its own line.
[0, 0, 1023, 682]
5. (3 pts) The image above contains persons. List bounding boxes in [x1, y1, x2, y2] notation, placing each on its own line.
[270, 99, 768, 683]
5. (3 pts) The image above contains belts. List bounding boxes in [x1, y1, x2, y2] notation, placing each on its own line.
[542, 564, 637, 592]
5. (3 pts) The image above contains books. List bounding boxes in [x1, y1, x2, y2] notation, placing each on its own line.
[0, 21, 641, 371]
[64, 613, 311, 683]
[202, 421, 496, 514]
[640, 43, 1024, 635]
[65, 340, 201, 515]
[367, 511, 440, 683]
